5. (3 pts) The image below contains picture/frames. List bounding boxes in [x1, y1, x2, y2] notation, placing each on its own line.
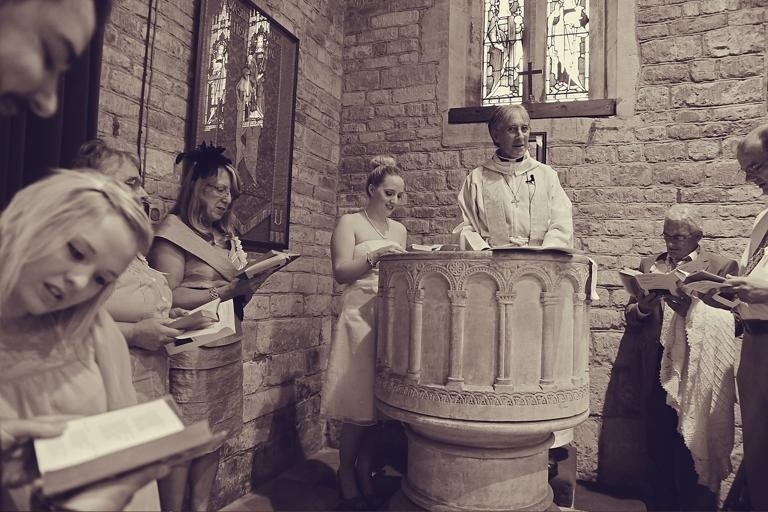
[184, 0, 300, 250]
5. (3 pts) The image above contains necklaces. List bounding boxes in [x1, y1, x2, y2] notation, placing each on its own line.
[359, 206, 390, 240]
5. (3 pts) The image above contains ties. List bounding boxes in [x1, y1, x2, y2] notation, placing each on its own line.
[739, 231, 768, 278]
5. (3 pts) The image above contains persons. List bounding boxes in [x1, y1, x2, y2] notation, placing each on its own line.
[0, 167, 163, 511]
[621, 203, 742, 511]
[0, 0, 170, 511]
[317, 154, 407, 510]
[453, 105, 575, 475]
[149, 142, 275, 511]
[699, 123, 768, 511]
[68, 136, 189, 403]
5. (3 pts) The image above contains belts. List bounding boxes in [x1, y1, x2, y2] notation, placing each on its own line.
[740, 320, 768, 335]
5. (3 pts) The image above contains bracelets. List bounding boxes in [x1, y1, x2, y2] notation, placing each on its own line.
[208, 287, 219, 301]
[365, 252, 378, 268]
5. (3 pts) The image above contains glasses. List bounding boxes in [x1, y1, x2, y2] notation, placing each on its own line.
[733, 163, 764, 180]
[660, 231, 694, 243]
[206, 182, 233, 199]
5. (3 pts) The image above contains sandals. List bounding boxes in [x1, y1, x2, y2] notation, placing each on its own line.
[338, 467, 383, 510]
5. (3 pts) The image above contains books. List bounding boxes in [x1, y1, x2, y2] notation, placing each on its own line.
[621, 258, 664, 297]
[403, 243, 445, 252]
[675, 269, 743, 309]
[234, 248, 301, 282]
[486, 241, 587, 256]
[164, 297, 222, 331]
[636, 257, 703, 303]
[28, 393, 230, 506]
